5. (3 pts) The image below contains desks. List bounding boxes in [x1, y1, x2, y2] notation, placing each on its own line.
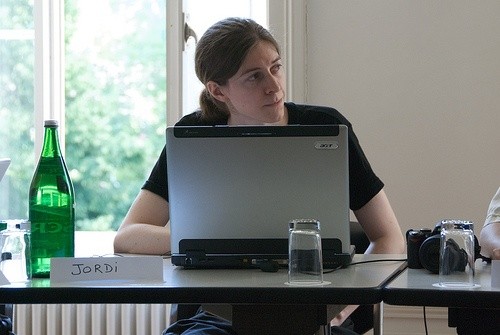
[383, 257, 500, 335]
[0, 253, 410, 335]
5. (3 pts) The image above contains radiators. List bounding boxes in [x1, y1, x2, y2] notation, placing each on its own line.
[12, 304, 178, 335]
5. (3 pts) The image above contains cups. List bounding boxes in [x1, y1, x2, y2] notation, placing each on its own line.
[440, 219, 474, 287]
[0, 219, 32, 288]
[289, 220, 324, 284]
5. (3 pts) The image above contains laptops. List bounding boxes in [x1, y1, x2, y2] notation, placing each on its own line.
[166, 124, 356, 270]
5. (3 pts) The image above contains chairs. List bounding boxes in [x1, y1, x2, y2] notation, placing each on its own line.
[170, 221, 383, 335]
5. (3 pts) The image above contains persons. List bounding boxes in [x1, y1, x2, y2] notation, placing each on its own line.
[114, 19, 404, 335]
[479, 187, 500, 260]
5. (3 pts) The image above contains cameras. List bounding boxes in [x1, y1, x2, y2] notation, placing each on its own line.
[405, 220, 481, 274]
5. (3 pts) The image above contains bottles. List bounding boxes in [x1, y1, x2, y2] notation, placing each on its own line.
[24, 120, 74, 276]
